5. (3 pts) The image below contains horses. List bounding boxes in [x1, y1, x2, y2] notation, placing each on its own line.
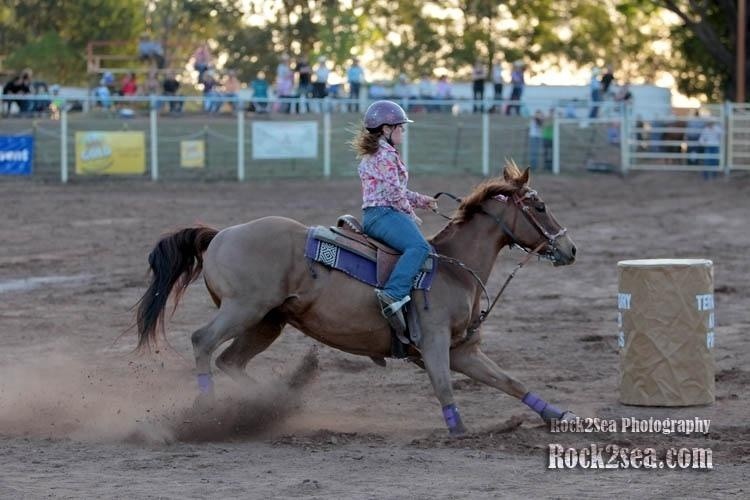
[112, 155, 583, 441]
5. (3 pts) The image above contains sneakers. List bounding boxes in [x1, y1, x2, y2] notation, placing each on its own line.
[377, 291, 407, 331]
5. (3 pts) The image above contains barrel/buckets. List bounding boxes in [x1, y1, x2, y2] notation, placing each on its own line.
[617, 257, 718, 405]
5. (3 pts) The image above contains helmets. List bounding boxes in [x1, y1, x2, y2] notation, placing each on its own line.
[365, 100, 415, 130]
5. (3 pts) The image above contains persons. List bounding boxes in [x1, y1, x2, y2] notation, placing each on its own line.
[0, 32, 724, 181]
[344, 99, 441, 337]
